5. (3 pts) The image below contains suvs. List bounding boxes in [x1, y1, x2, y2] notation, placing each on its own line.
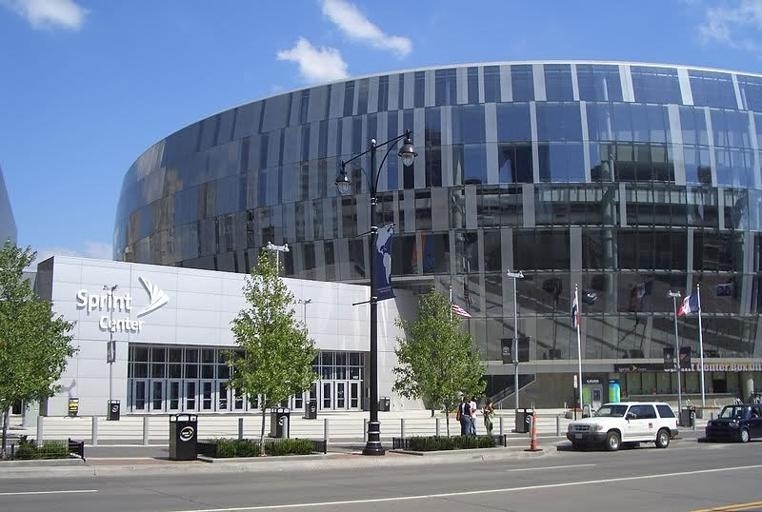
[705, 404, 762, 443]
[566, 402, 680, 451]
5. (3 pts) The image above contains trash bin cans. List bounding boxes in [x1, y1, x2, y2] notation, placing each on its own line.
[270, 406, 290, 438]
[681, 406, 695, 427]
[305, 401, 317, 419]
[380, 396, 390, 411]
[515, 407, 533, 433]
[107, 400, 120, 420]
[169, 414, 198, 461]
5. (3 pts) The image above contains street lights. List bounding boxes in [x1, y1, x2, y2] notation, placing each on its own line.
[334, 130, 419, 456]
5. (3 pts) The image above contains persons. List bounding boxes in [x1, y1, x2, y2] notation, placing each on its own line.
[468, 394, 478, 435]
[480, 397, 496, 436]
[459, 394, 473, 437]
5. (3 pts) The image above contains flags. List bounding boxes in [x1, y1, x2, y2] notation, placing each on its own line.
[570, 296, 580, 328]
[451, 295, 473, 319]
[676, 291, 698, 319]
